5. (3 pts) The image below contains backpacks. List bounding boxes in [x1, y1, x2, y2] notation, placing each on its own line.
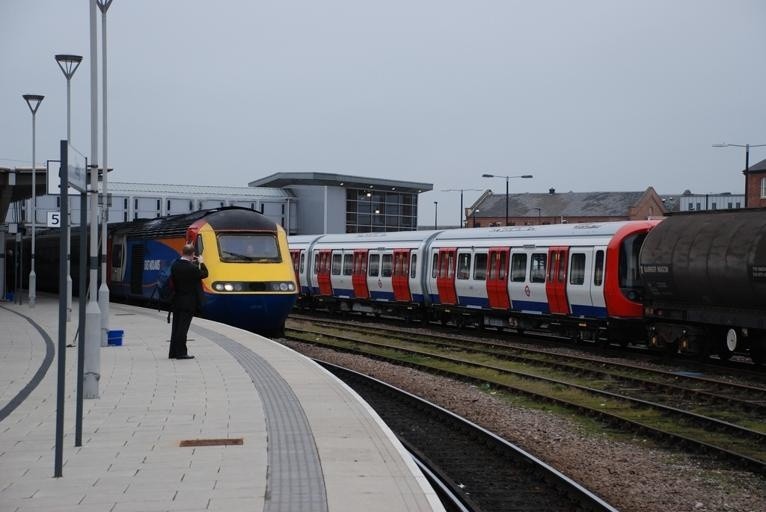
[151, 254, 181, 311]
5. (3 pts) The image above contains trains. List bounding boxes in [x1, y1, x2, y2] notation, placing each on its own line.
[289, 207, 766, 360]
[12, 206, 298, 328]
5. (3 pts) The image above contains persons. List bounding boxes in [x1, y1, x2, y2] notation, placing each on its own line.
[167, 244, 208, 360]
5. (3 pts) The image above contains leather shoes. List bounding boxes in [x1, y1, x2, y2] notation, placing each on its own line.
[169, 354, 195, 359]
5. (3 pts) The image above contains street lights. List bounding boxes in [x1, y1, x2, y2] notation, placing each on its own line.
[55, 54, 83, 325]
[23, 95, 45, 307]
[95, 0, 113, 347]
[481, 173, 533, 226]
[440, 189, 483, 228]
[712, 142, 766, 208]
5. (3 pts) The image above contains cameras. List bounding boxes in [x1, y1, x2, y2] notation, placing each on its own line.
[194, 257, 198, 262]
